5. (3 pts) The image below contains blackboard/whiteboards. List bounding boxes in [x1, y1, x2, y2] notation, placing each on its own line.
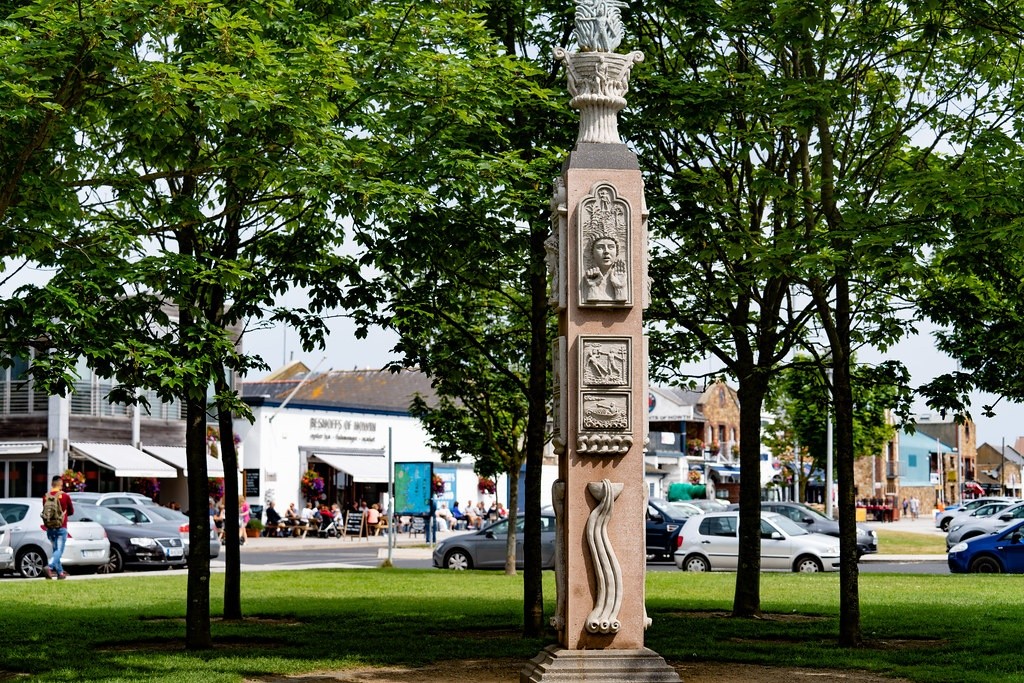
[344, 510, 368, 538]
[244, 468, 260, 497]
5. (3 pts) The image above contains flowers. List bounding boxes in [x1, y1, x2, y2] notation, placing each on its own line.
[478, 478, 497, 495]
[62, 468, 86, 494]
[301, 470, 326, 495]
[710, 439, 720, 455]
[689, 470, 701, 485]
[686, 437, 705, 455]
[208, 475, 224, 502]
[139, 474, 159, 501]
[206, 425, 243, 445]
[432, 475, 445, 495]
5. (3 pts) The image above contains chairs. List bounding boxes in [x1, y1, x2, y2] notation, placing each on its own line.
[131, 517, 144, 523]
[709, 519, 723, 531]
[5, 510, 28, 523]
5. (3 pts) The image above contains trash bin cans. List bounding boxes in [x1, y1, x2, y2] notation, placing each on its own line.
[249, 505, 263, 524]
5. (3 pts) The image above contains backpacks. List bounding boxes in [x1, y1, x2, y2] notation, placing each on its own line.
[42, 491, 67, 528]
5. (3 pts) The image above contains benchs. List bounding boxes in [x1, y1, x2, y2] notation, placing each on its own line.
[215, 524, 396, 535]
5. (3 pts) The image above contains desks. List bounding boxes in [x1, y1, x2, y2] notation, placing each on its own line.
[373, 515, 394, 536]
[288, 516, 321, 538]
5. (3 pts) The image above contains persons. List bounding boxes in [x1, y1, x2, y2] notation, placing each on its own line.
[902, 496, 920, 520]
[586, 233, 627, 302]
[862, 495, 883, 506]
[43, 475, 74, 580]
[579, 8, 616, 53]
[351, 500, 383, 537]
[588, 349, 625, 377]
[595, 56, 608, 96]
[239, 495, 251, 545]
[266, 501, 281, 526]
[285, 503, 343, 537]
[435, 500, 505, 531]
[209, 497, 225, 542]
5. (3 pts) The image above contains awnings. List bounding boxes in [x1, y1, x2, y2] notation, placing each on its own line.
[144, 445, 241, 477]
[788, 462, 824, 486]
[70, 442, 177, 477]
[314, 453, 394, 484]
[966, 482, 985, 495]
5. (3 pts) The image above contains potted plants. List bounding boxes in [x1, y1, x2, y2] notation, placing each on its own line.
[246, 517, 266, 537]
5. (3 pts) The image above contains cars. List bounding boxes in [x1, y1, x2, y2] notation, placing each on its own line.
[935, 496, 1024, 574]
[646, 498, 730, 562]
[673, 502, 879, 573]
[65, 502, 188, 574]
[433, 506, 556, 571]
[0, 497, 110, 578]
[64, 492, 220, 568]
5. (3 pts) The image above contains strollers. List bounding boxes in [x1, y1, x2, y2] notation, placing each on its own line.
[318, 509, 341, 538]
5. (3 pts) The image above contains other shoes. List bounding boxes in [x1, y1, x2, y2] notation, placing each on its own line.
[44, 566, 52, 579]
[58, 572, 67, 579]
